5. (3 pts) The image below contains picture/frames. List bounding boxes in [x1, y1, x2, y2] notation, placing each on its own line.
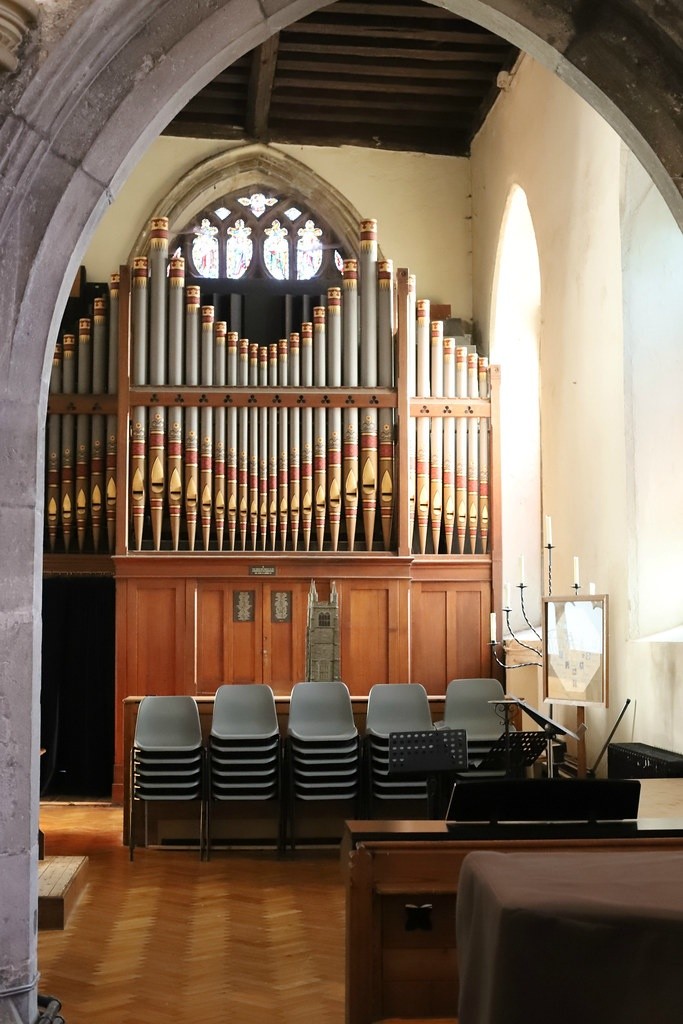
[541, 594, 609, 708]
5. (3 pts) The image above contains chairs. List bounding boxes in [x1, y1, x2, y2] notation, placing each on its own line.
[128, 678, 517, 862]
[445, 776, 639, 820]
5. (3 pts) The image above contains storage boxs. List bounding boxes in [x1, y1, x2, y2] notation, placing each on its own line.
[608, 743, 683, 779]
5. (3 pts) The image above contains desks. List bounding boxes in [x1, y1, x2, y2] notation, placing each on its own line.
[339, 820, 683, 1024]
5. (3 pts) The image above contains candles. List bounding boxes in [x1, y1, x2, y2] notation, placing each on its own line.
[589, 582, 596, 595]
[573, 556, 579, 587]
[504, 582, 510, 609]
[490, 612, 496, 644]
[519, 553, 524, 583]
[545, 514, 552, 547]
